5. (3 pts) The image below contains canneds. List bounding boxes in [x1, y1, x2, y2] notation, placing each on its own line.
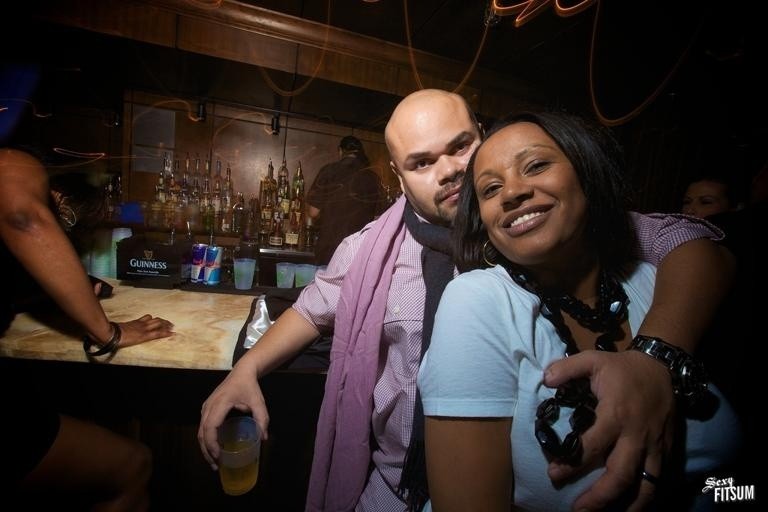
[203, 245, 223, 285]
[190, 243, 208, 283]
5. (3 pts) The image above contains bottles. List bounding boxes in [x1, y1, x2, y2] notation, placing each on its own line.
[149, 150, 314, 252]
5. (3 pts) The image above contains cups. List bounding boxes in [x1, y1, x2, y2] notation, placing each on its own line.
[233, 258, 256, 290]
[276, 262, 327, 289]
[214, 416, 262, 495]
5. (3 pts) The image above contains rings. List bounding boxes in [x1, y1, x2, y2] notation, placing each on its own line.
[634, 466, 665, 486]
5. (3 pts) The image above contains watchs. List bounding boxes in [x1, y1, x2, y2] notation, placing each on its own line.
[626, 332, 711, 404]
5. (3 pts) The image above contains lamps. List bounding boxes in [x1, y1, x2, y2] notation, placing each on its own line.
[196, 100, 207, 123]
[271, 112, 280, 136]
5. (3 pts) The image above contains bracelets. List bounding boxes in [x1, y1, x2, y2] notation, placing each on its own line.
[82, 321, 122, 356]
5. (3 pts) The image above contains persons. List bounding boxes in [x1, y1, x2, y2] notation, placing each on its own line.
[678, 159, 739, 219]
[701, 138, 768, 422]
[417, 106, 767, 512]
[196, 86, 494, 512]
[0, 127, 175, 512]
[303, 135, 383, 266]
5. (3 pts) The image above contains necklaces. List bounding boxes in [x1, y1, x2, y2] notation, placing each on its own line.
[524, 261, 633, 463]
